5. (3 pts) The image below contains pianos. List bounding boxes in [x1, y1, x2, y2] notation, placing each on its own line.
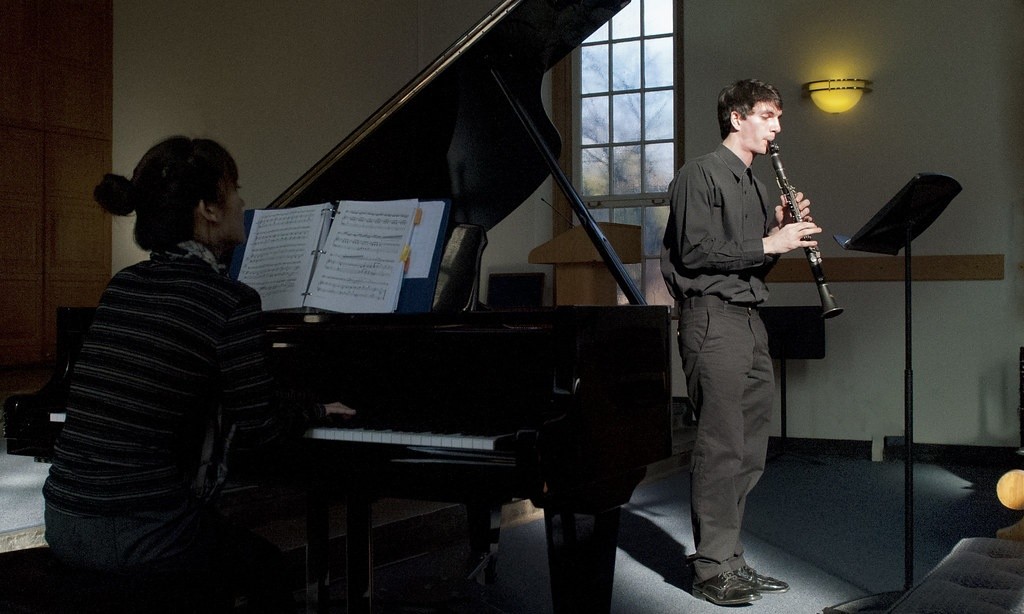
[0, 0, 701, 613]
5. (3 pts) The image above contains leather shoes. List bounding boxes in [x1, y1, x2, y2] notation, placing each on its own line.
[692, 570, 763, 605]
[733, 564, 790, 593]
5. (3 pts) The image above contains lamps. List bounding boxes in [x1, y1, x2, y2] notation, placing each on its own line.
[801, 79, 873, 114]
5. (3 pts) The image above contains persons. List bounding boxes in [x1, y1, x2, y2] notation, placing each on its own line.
[42, 134, 358, 614]
[659, 79, 822, 606]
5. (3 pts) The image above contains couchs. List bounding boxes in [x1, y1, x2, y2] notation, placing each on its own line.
[885, 538, 1024, 614]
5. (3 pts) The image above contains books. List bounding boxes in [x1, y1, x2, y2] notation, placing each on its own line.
[237, 198, 422, 315]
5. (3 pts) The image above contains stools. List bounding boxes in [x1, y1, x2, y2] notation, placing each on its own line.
[0, 549, 241, 614]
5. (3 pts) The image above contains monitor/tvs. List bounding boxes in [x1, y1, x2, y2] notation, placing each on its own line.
[483, 263, 555, 308]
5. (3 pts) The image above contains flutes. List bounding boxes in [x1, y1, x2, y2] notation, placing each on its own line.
[767, 142, 846, 320]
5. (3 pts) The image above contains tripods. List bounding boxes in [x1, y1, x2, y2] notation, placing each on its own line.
[754, 306, 829, 468]
[824, 173, 962, 614]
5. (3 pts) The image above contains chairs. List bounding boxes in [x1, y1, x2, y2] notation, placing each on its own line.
[486, 272, 545, 310]
